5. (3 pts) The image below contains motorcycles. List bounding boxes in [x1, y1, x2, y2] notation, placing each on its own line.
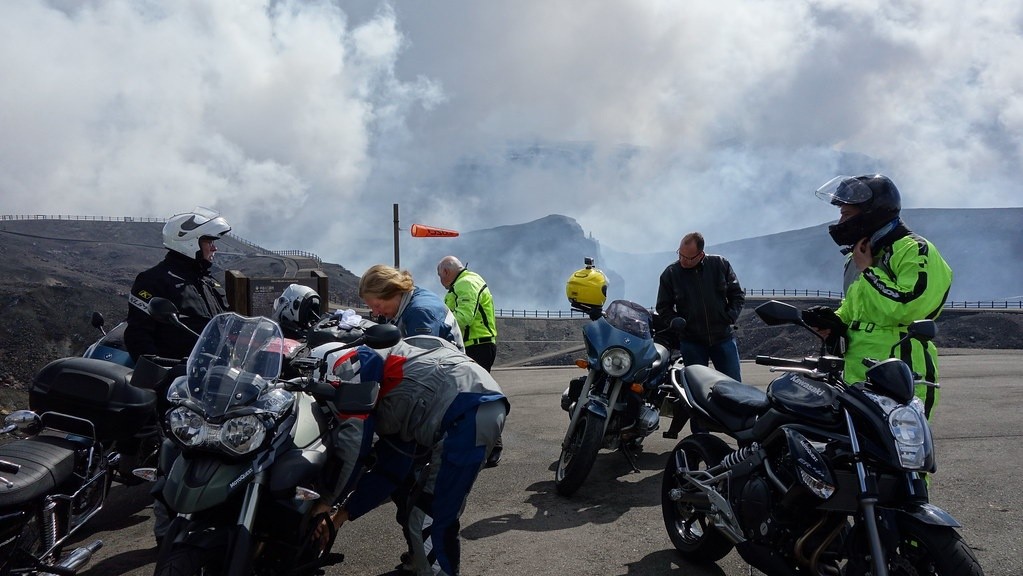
[547, 256, 688, 501]
[81, 312, 179, 384]
[145, 283, 401, 576]
[662, 296, 989, 576]
[0, 356, 164, 576]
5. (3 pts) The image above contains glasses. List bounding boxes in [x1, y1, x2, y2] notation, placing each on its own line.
[676, 248, 702, 260]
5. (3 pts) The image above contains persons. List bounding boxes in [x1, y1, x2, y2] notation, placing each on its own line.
[303, 334, 511, 574]
[814, 174, 953, 488]
[438, 255, 499, 372]
[129, 207, 236, 552]
[652, 232, 744, 383]
[356, 263, 463, 355]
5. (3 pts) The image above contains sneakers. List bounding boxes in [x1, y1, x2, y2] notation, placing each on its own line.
[486, 434, 502, 465]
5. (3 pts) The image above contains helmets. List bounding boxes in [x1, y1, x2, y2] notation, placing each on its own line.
[274, 283, 322, 330]
[814, 173, 902, 248]
[566, 266, 610, 307]
[161, 206, 231, 258]
